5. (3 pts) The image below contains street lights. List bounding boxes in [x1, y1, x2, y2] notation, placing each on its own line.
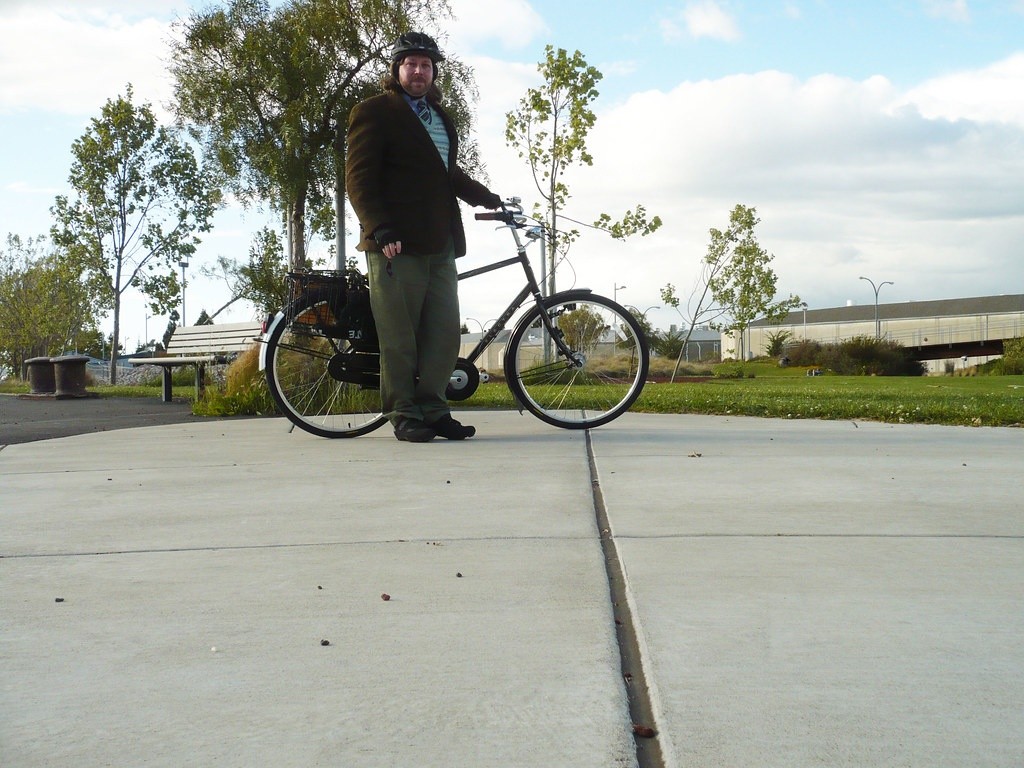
[124, 337, 130, 356]
[465, 318, 500, 367]
[802, 308, 808, 342]
[145, 314, 152, 350]
[623, 304, 661, 324]
[859, 276, 894, 340]
[614, 285, 627, 354]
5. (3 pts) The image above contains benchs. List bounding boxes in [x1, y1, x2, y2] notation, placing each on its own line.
[127, 321, 265, 404]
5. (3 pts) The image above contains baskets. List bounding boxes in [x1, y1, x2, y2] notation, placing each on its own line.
[285, 269, 370, 340]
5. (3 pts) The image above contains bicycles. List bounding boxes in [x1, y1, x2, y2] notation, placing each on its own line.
[252, 196, 650, 441]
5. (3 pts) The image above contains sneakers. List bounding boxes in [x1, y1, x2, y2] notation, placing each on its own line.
[394, 418, 437, 443]
[429, 413, 476, 441]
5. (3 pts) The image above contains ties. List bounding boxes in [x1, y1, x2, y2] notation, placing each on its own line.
[416, 100, 432, 126]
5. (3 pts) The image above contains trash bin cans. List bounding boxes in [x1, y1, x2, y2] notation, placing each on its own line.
[51, 355, 91, 397]
[21, 356, 55, 395]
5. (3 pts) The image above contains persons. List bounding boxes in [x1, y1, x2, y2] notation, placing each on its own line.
[345, 33, 501, 442]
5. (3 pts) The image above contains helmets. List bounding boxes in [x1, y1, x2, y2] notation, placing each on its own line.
[390, 31, 446, 62]
